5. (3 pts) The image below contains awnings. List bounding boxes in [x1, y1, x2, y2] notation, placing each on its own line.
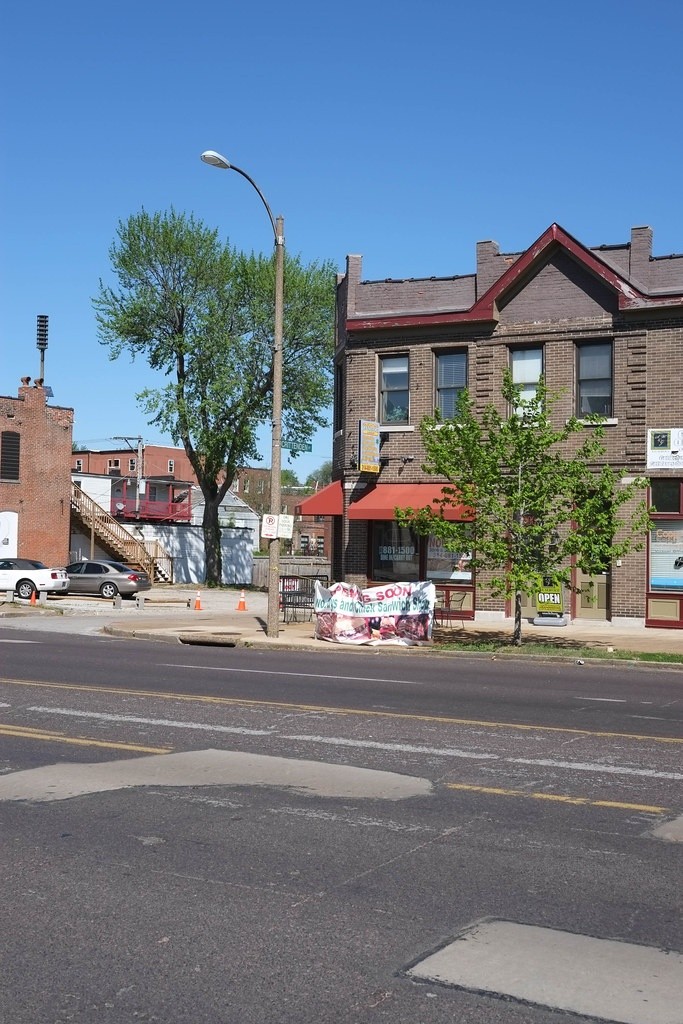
[347, 484, 476, 521]
[294, 479, 343, 515]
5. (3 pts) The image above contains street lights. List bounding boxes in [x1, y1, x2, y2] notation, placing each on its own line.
[201, 150, 296, 636]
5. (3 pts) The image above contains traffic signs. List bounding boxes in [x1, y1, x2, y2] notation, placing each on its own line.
[261, 513, 278, 539]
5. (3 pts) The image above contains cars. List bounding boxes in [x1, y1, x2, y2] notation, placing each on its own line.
[57, 560, 152, 598]
[0, 558, 70, 599]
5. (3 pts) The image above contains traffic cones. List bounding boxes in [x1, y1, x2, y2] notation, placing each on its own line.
[235, 589, 249, 612]
[194, 589, 203, 610]
[29, 591, 37, 604]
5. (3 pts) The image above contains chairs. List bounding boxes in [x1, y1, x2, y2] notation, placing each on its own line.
[437, 590, 466, 630]
